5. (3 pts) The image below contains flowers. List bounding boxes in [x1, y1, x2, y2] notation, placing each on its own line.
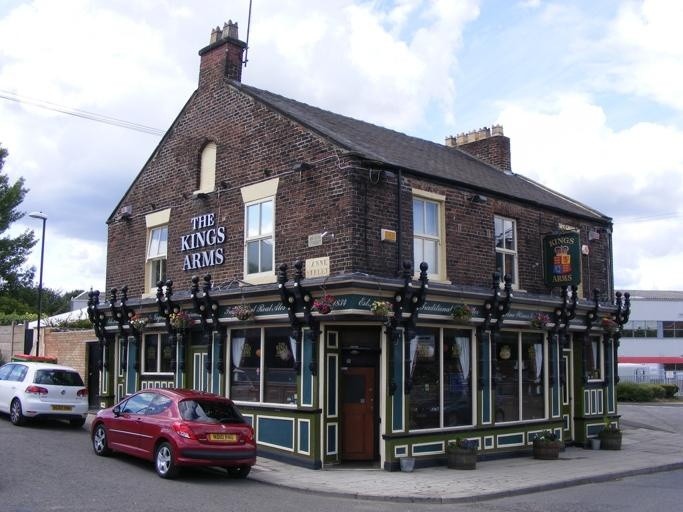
[228, 304, 257, 320]
[452, 303, 478, 322]
[168, 311, 194, 328]
[310, 288, 336, 314]
[531, 312, 550, 328]
[130, 314, 148, 329]
[371, 300, 393, 317]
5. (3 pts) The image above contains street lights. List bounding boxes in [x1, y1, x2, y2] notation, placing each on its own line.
[29, 211, 48, 356]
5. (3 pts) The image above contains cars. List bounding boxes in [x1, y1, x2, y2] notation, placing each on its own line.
[0, 361, 89, 427]
[413, 391, 504, 429]
[231, 369, 257, 402]
[91, 387, 257, 480]
